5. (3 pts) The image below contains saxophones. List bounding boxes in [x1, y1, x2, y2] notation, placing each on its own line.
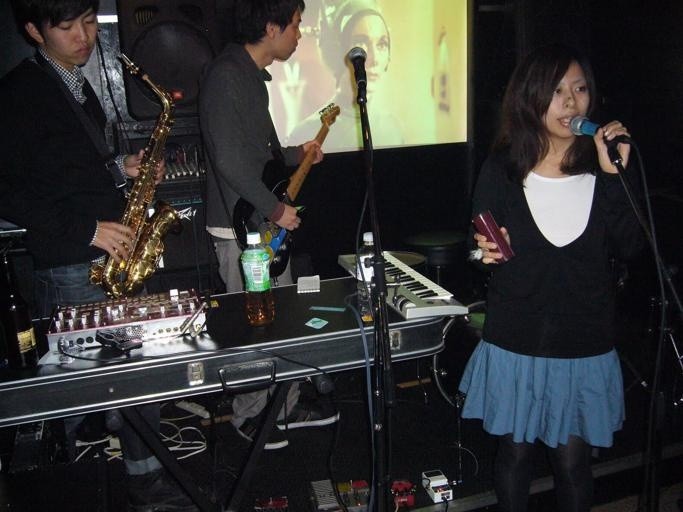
[89, 53, 183, 298]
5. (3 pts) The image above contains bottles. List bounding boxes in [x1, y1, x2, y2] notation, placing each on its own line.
[0, 250, 40, 371]
[240, 231, 276, 328]
[355, 231, 376, 324]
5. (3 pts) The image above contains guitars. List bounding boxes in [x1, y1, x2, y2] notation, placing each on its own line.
[233, 103, 340, 278]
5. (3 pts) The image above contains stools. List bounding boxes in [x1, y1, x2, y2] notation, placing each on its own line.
[406, 226, 468, 286]
[358, 250, 422, 406]
[433, 300, 497, 482]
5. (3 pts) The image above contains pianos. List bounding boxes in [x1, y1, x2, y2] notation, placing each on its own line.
[338, 251, 469, 318]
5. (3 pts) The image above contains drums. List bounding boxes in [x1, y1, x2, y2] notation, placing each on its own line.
[413, 231, 464, 268]
[433, 300, 486, 411]
[392, 251, 427, 275]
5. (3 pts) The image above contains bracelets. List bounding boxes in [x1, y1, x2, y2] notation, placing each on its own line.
[88, 220, 97, 246]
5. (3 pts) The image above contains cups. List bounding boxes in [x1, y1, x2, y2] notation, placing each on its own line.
[471, 208, 515, 265]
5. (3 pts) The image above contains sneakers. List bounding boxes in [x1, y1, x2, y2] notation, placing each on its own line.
[277, 404, 341, 430]
[238, 416, 288, 450]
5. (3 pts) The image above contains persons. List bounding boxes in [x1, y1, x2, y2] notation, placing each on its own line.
[0, 0, 199, 509]
[457, 42, 644, 510]
[197, 0, 344, 449]
[283, 1, 413, 153]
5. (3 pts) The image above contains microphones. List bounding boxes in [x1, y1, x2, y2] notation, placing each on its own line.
[348, 48, 367, 106]
[569, 115, 631, 147]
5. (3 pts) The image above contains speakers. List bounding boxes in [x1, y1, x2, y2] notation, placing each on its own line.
[116, 1, 216, 119]
